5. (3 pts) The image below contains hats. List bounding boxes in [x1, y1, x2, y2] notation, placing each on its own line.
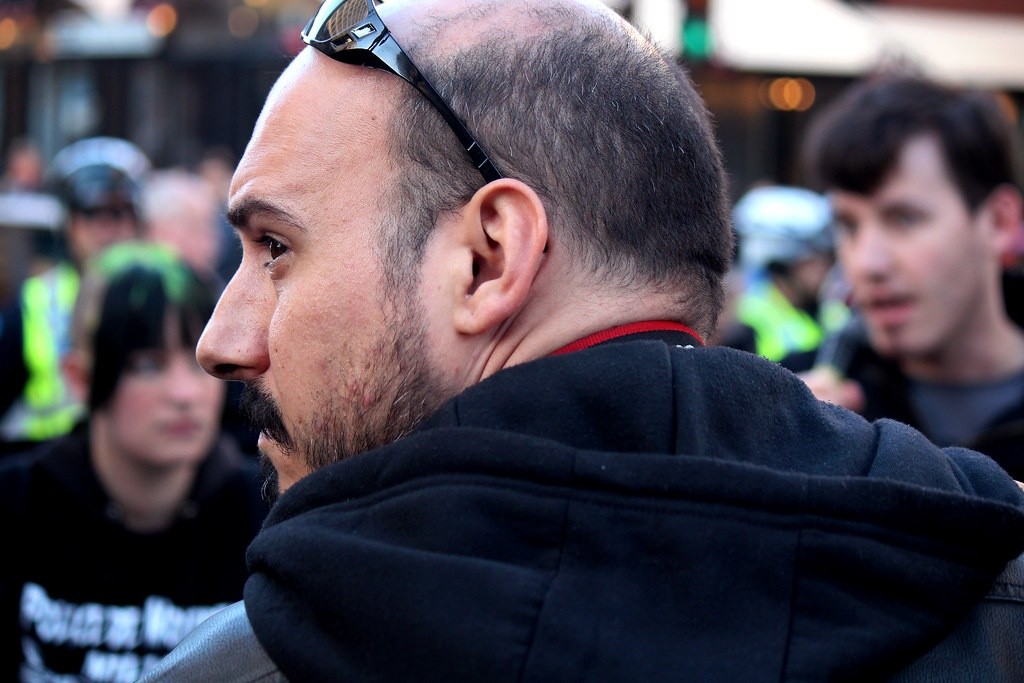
[45, 137, 153, 212]
[0, 187, 63, 229]
[736, 187, 834, 271]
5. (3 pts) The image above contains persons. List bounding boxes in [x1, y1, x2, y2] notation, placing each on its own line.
[0, 131, 280, 682]
[129, 0, 1024, 683]
[710, 58, 1024, 483]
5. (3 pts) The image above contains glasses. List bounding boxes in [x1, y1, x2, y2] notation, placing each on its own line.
[299, 0, 549, 256]
